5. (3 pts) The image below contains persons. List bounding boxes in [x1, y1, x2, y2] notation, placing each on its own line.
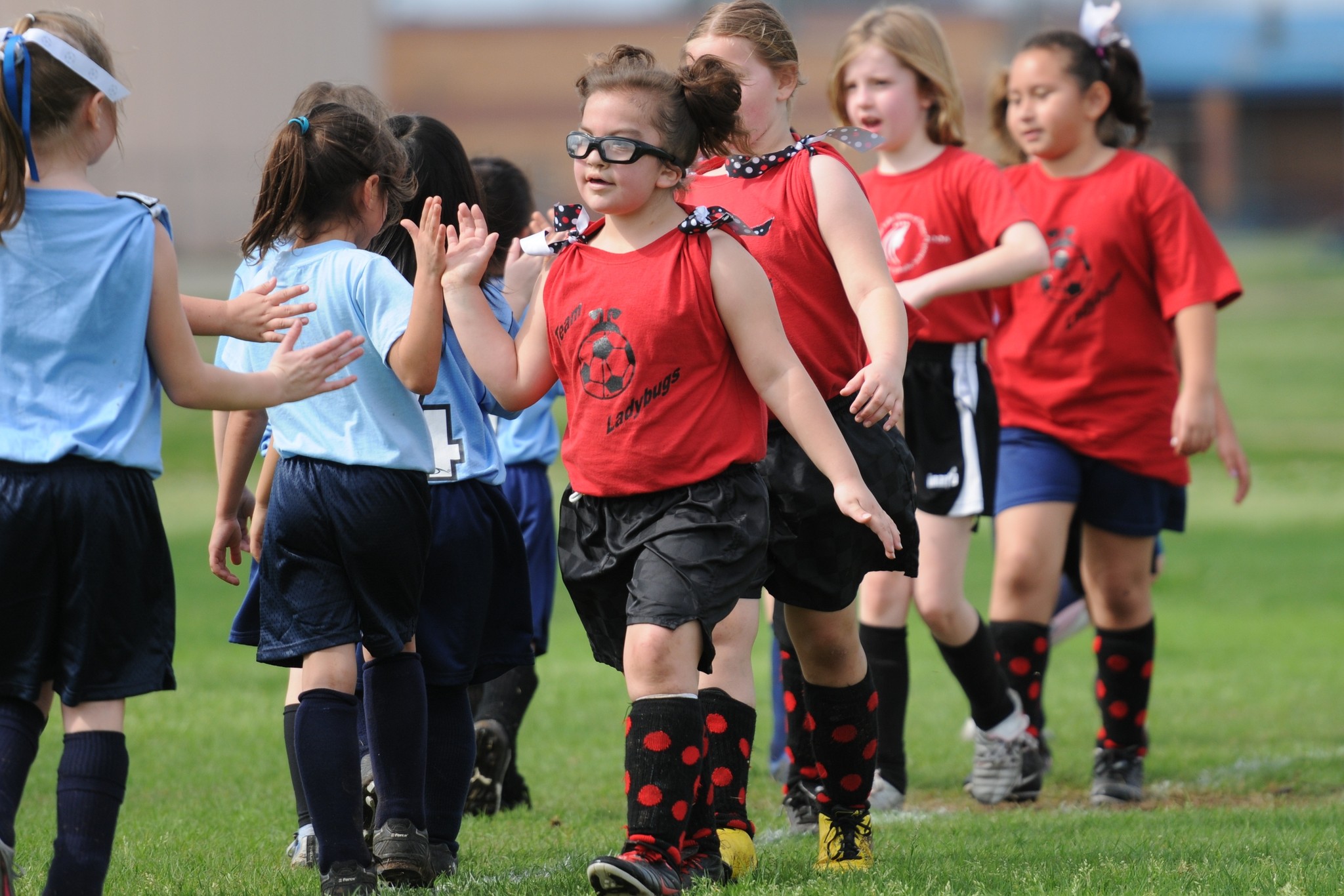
[206, 102, 454, 896]
[0, 12, 366, 894]
[462, 152, 572, 826]
[365, 115, 533, 876]
[667, 1, 923, 884]
[975, 31, 1254, 808]
[821, 7, 1060, 819]
[211, 71, 440, 871]
[169, 271, 321, 346]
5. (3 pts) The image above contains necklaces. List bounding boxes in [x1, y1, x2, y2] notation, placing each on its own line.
[440, 37, 917, 895]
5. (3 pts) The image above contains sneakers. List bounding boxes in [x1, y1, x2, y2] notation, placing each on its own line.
[816, 809, 874, 877]
[286, 824, 320, 867]
[357, 753, 378, 854]
[315, 859, 378, 896]
[963, 734, 1048, 803]
[585, 841, 688, 896]
[711, 826, 758, 881]
[781, 784, 820, 836]
[866, 767, 906, 812]
[462, 717, 512, 817]
[672, 835, 729, 889]
[371, 818, 437, 887]
[1091, 747, 1145, 804]
[960, 688, 1030, 804]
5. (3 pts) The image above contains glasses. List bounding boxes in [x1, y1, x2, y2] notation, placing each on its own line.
[565, 130, 686, 178]
